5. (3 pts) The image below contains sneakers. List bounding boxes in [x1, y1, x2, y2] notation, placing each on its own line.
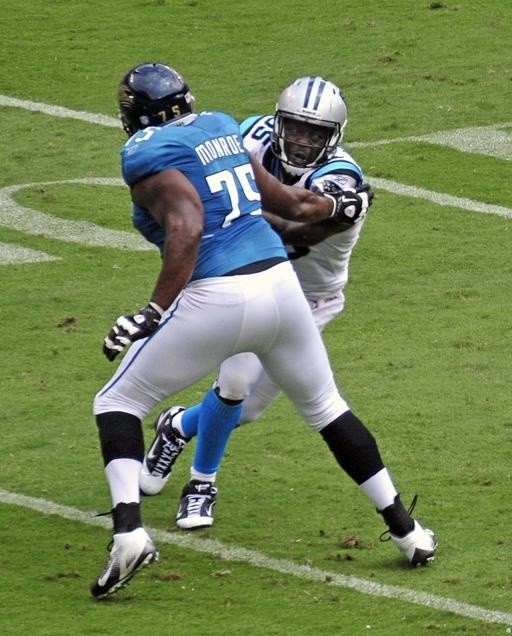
[175, 478, 218, 531]
[387, 519, 439, 569]
[140, 405, 193, 497]
[91, 525, 159, 601]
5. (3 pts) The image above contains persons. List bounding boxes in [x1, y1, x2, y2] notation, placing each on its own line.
[89, 59, 443, 601]
[136, 73, 370, 531]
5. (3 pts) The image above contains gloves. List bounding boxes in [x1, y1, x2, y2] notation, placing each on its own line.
[102, 300, 167, 362]
[324, 182, 375, 225]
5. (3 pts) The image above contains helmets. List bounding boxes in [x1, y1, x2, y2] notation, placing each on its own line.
[114, 60, 196, 135]
[266, 75, 349, 176]
